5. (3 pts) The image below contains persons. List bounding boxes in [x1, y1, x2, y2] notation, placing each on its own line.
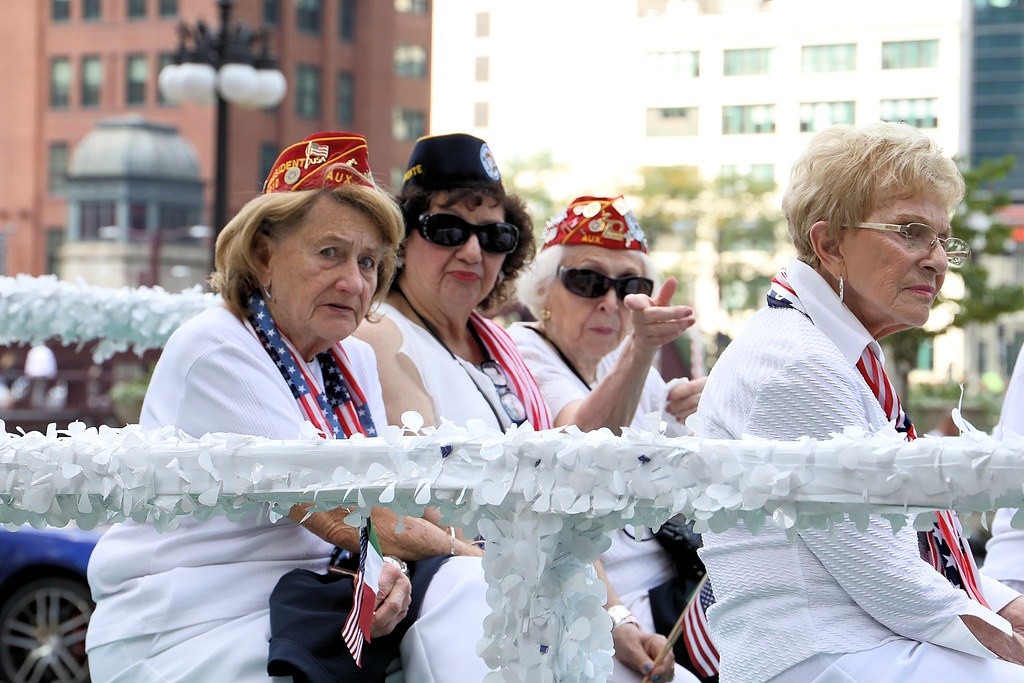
[81, 128, 1024, 682]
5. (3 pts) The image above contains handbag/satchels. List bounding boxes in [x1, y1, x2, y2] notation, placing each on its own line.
[648, 513, 706, 582]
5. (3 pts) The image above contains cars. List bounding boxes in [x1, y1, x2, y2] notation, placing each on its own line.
[0, 518, 101, 683]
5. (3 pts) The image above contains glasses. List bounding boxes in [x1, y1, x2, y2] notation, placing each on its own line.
[834, 219, 971, 268]
[481, 359, 526, 422]
[555, 266, 654, 302]
[411, 212, 520, 255]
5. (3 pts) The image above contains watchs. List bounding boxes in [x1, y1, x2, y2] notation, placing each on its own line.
[385, 554, 410, 577]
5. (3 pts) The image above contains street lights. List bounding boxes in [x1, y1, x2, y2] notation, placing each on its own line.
[156, 0, 287, 279]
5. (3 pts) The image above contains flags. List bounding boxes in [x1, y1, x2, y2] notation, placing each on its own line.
[338, 506, 382, 670]
[681, 575, 724, 677]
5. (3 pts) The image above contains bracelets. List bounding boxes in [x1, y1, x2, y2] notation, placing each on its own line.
[607, 604, 640, 630]
[445, 525, 455, 559]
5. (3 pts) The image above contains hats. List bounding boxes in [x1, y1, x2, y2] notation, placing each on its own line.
[23, 347, 58, 379]
[396, 133, 505, 202]
[261, 132, 376, 195]
[542, 194, 648, 254]
